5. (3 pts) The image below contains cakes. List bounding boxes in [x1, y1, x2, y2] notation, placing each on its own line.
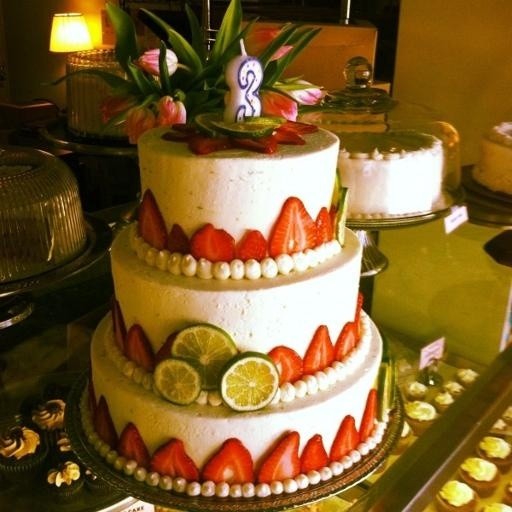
[0, 54, 512, 512]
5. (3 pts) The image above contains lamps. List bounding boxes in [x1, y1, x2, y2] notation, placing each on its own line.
[48, 12, 93, 108]
